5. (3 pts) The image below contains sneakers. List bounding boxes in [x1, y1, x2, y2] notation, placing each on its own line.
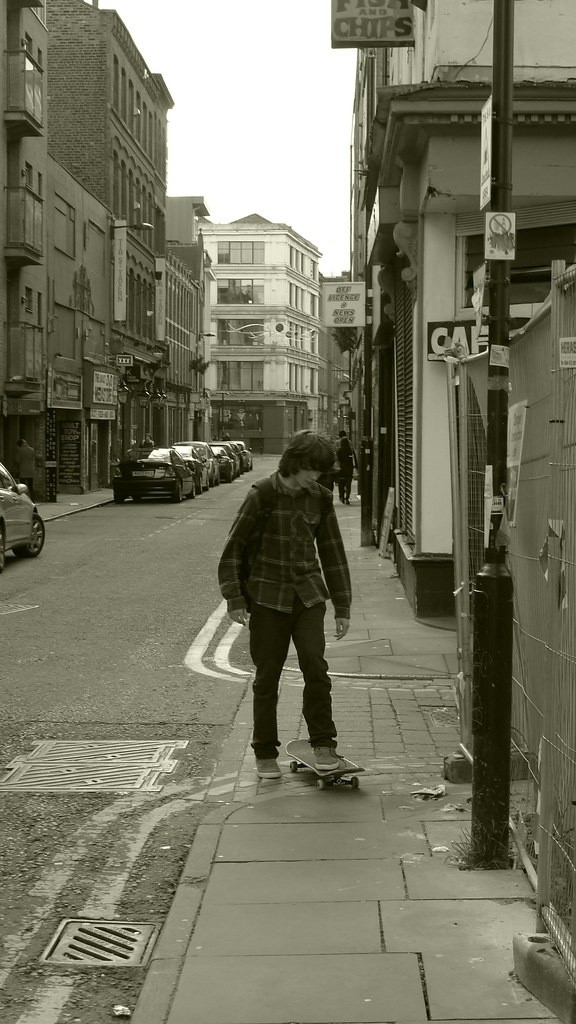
[313, 746, 347, 770]
[255, 757, 282, 779]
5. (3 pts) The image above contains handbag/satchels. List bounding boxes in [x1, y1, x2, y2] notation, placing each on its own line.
[351, 448, 356, 466]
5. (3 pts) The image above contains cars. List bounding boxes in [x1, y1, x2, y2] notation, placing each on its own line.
[112, 446, 197, 503]
[176, 440, 221, 489]
[0, 462, 45, 573]
[170, 445, 212, 493]
[207, 438, 253, 486]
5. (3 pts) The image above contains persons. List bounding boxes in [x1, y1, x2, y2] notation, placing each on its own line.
[328, 431, 357, 504]
[15, 439, 35, 503]
[141, 434, 154, 447]
[217, 430, 352, 779]
[223, 433, 231, 441]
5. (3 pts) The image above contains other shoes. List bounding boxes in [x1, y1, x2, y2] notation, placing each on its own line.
[344, 498, 349, 504]
[339, 497, 344, 503]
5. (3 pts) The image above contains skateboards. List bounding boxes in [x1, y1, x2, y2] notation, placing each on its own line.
[284, 739, 366, 790]
